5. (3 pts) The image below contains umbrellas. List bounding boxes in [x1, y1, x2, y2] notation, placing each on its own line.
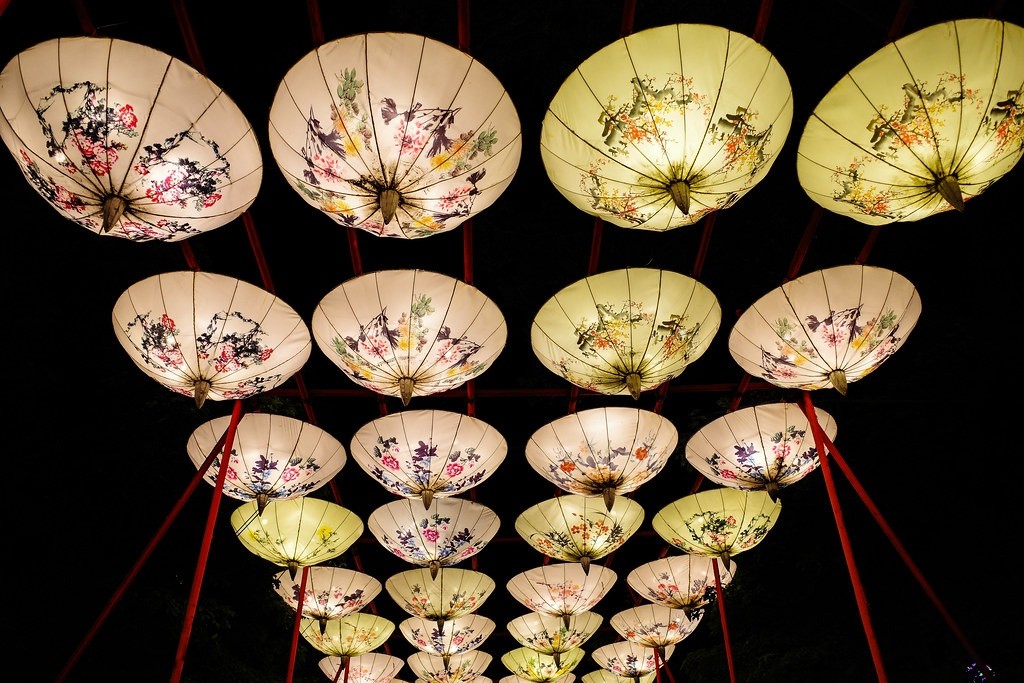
[526, 407, 679, 513]
[350, 409, 507, 511]
[540, 24, 793, 232]
[0, 36, 263, 242]
[728, 264, 922, 397]
[685, 403, 838, 504]
[532, 268, 722, 401]
[111, 270, 312, 409]
[186, 414, 346, 517]
[267, 32, 523, 241]
[230, 488, 781, 683]
[311, 270, 507, 406]
[798, 18, 1024, 226]
